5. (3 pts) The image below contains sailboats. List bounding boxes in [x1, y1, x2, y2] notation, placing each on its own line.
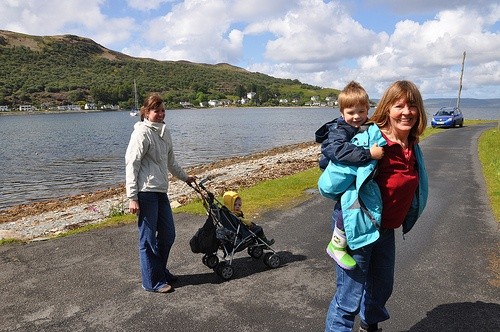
[130, 77, 140, 117]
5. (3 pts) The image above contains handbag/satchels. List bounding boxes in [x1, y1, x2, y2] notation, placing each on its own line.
[189, 216, 221, 254]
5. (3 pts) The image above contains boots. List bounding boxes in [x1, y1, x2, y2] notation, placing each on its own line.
[326, 227, 357, 271]
[259, 236, 275, 246]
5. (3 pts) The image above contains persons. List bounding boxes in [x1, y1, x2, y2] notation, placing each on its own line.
[317, 80, 429, 332]
[124, 96, 196, 293]
[315, 81, 384, 270]
[223, 191, 275, 249]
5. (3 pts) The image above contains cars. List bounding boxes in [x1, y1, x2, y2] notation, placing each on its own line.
[431, 107, 464, 129]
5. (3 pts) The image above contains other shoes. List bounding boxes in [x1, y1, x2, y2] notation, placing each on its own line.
[158, 284, 171, 293]
[171, 276, 178, 283]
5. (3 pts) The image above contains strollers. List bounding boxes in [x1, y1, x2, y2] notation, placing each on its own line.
[187, 176, 281, 280]
